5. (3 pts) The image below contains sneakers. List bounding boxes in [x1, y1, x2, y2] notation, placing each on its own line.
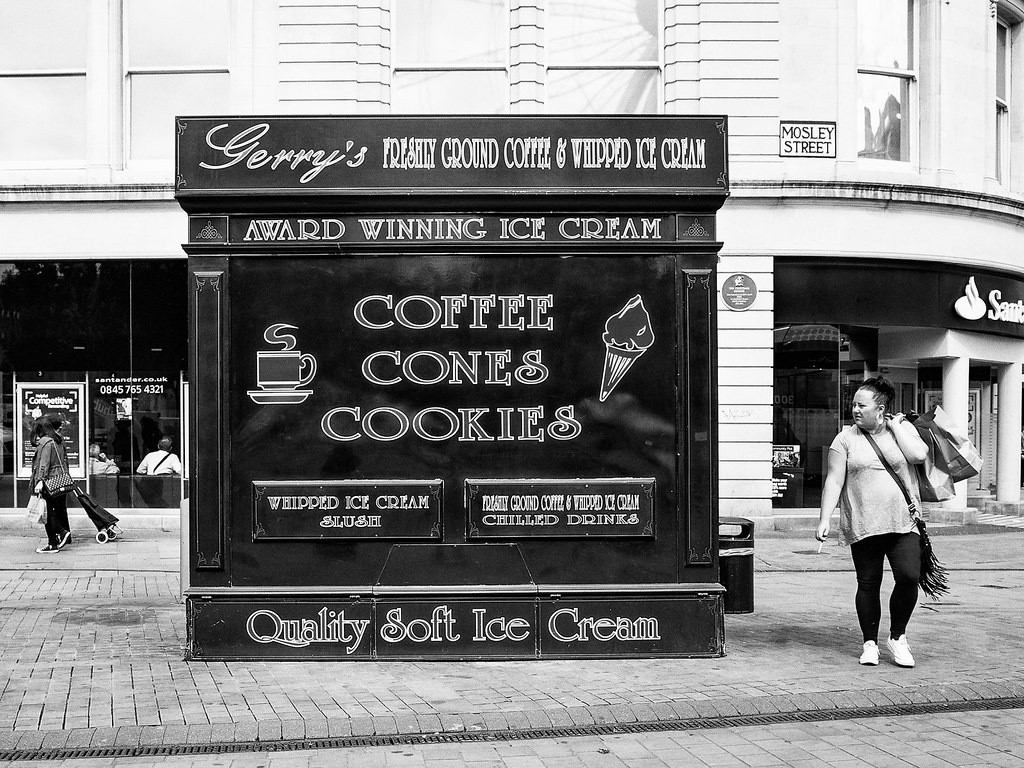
[886, 633, 916, 668]
[56, 529, 71, 548]
[859, 640, 880, 666]
[36, 544, 59, 554]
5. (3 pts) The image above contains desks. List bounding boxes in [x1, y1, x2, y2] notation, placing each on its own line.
[772, 467, 805, 509]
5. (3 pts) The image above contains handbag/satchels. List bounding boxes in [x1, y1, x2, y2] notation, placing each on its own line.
[914, 427, 956, 502]
[916, 519, 951, 603]
[44, 472, 75, 496]
[24, 495, 48, 525]
[913, 405, 984, 483]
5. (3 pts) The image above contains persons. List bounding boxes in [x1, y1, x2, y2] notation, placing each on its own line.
[136, 436, 181, 475]
[30, 414, 72, 553]
[816, 375, 929, 667]
[89, 444, 120, 475]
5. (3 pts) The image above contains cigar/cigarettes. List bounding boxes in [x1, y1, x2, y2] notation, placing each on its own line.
[817, 541, 822, 553]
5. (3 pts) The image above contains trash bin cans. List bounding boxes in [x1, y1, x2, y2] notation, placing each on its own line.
[716, 514, 757, 617]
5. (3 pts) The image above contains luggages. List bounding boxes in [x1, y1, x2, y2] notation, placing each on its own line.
[70, 480, 123, 544]
